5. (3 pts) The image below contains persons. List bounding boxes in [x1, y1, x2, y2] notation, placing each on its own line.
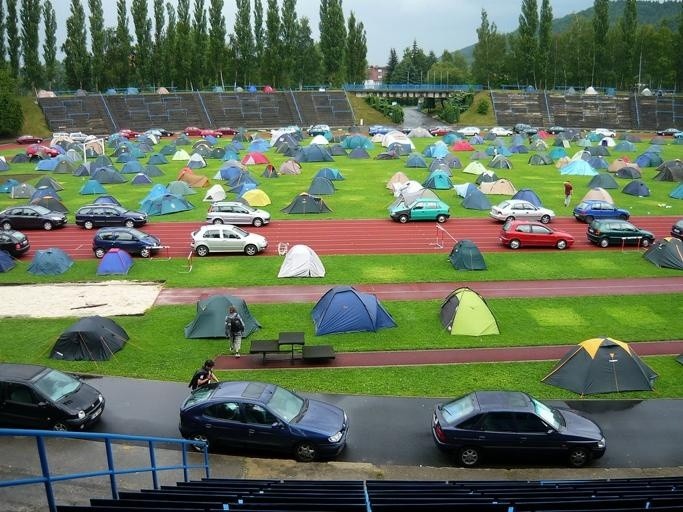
[187, 358, 221, 390]
[224, 305, 244, 359]
[656, 90, 673, 97]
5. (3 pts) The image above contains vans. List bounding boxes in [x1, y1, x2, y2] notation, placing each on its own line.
[0, 363, 105, 433]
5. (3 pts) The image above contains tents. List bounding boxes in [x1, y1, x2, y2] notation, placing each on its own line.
[0, 249, 16, 272]
[1, 122, 683, 215]
[274, 242, 327, 279]
[640, 236, 682, 272]
[46, 315, 129, 361]
[35, 85, 273, 98]
[522, 85, 655, 97]
[445, 240, 487, 272]
[309, 286, 398, 337]
[537, 335, 658, 395]
[95, 245, 135, 276]
[25, 247, 73, 276]
[182, 293, 261, 341]
[438, 284, 503, 337]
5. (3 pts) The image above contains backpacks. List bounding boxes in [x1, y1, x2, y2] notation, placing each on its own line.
[229, 314, 242, 332]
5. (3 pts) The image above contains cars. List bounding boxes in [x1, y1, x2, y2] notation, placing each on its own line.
[546, 126, 574, 135]
[572, 200, 630, 224]
[0, 199, 271, 257]
[14, 127, 97, 159]
[104, 123, 239, 142]
[595, 127, 615, 137]
[390, 199, 451, 223]
[457, 127, 480, 136]
[585, 218, 655, 248]
[428, 126, 455, 136]
[488, 200, 555, 224]
[669, 219, 682, 240]
[656, 128, 679, 136]
[306, 124, 330, 136]
[400, 128, 413, 135]
[368, 125, 391, 136]
[489, 123, 538, 136]
[179, 381, 349, 463]
[496, 219, 574, 250]
[429, 390, 606, 468]
[672, 132, 682, 138]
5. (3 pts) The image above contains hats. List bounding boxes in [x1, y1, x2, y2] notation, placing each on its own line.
[205, 360, 215, 366]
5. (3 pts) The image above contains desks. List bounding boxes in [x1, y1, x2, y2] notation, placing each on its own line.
[248, 340, 279, 366]
[302, 345, 335, 365]
[278, 332, 305, 365]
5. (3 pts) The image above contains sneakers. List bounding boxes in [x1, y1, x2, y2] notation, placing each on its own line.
[230, 347, 240, 358]
